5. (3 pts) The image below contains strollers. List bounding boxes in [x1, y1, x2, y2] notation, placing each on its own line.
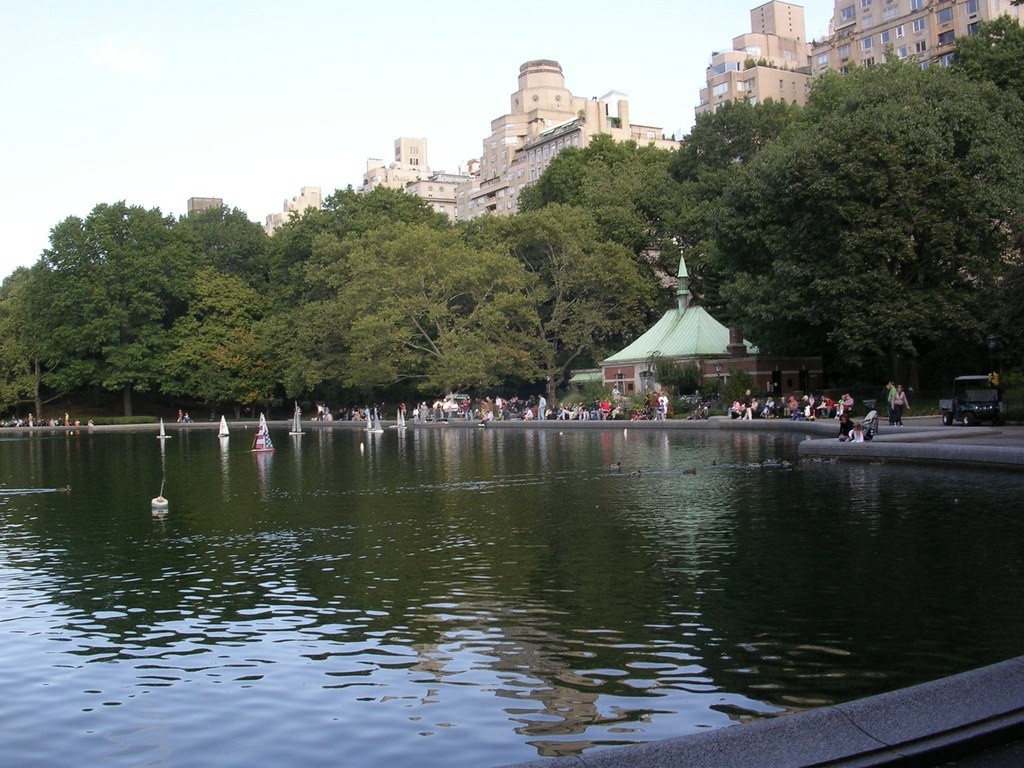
[861, 411, 880, 440]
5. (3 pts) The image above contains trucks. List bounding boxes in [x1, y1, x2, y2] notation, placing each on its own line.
[938, 375, 1004, 428]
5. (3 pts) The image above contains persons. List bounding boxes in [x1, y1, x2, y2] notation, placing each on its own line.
[28, 413, 33, 427]
[177, 409, 191, 423]
[612, 382, 620, 402]
[641, 390, 670, 419]
[886, 380, 910, 427]
[64, 413, 69, 426]
[730, 389, 855, 420]
[317, 402, 330, 421]
[837, 415, 867, 443]
[400, 394, 626, 419]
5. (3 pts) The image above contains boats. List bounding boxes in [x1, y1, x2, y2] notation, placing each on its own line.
[151, 481, 169, 508]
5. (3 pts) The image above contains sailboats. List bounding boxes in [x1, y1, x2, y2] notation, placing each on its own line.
[217, 415, 230, 437]
[389, 408, 407, 430]
[288, 400, 306, 435]
[363, 408, 384, 433]
[251, 412, 275, 452]
[156, 417, 172, 438]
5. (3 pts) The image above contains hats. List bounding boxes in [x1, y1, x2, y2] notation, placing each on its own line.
[802, 395, 808, 400]
